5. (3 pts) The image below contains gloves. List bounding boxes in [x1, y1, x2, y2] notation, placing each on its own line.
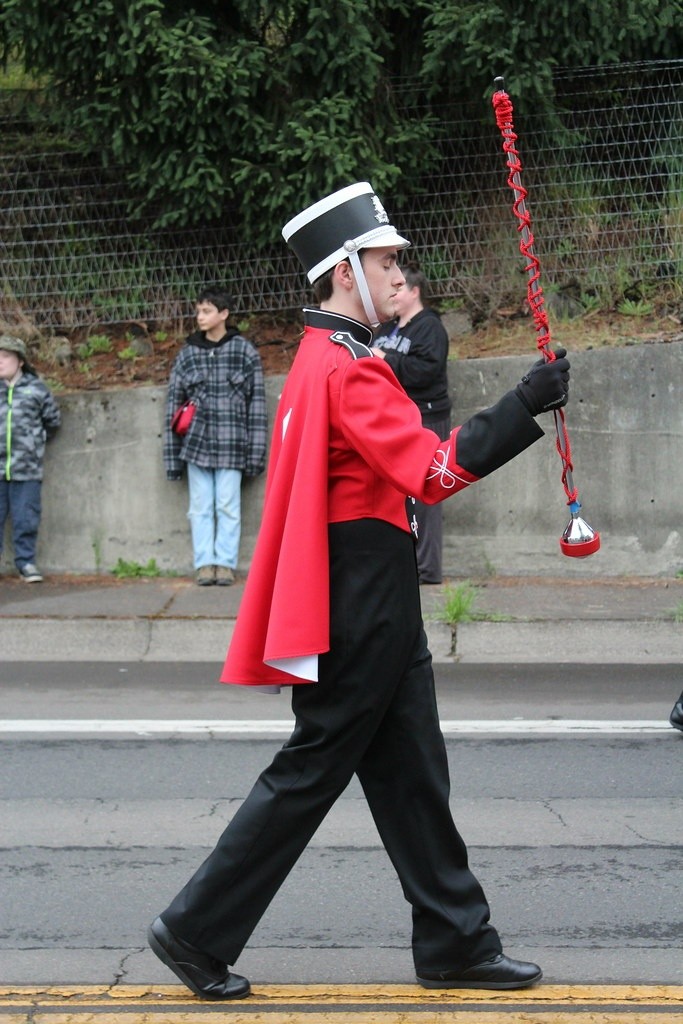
[517, 349, 570, 413]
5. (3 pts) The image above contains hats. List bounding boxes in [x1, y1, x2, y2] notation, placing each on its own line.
[282, 182, 410, 286]
[1, 334, 36, 375]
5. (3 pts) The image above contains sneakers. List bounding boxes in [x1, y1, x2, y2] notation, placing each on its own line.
[417, 950, 543, 989]
[196, 563, 235, 586]
[17, 562, 44, 582]
[149, 916, 252, 1001]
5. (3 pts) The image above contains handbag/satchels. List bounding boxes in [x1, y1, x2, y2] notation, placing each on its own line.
[169, 398, 196, 434]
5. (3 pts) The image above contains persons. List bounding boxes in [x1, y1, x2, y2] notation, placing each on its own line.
[670, 690, 683, 731]
[0, 334, 61, 582]
[144, 180, 570, 999]
